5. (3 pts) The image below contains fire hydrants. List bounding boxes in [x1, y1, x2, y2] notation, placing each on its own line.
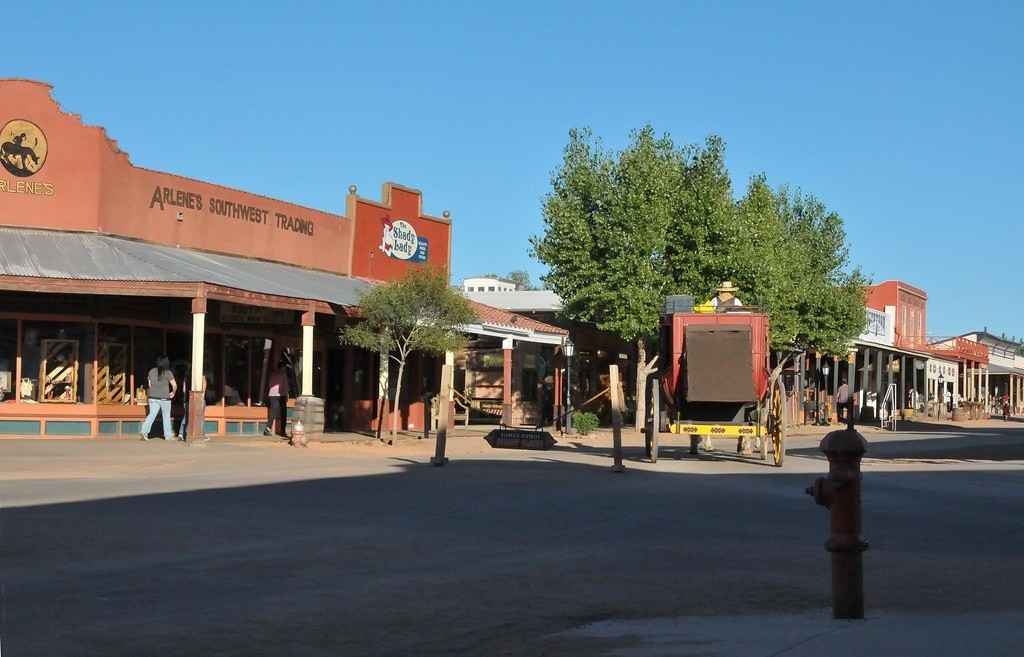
[290, 420, 305, 448]
[803, 428, 871, 622]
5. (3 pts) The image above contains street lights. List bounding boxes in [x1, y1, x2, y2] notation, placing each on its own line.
[821, 361, 831, 426]
[562, 337, 575, 435]
[938, 375, 944, 421]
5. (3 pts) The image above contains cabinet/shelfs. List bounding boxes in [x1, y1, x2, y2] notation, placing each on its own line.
[39, 338, 79, 404]
[92, 340, 125, 405]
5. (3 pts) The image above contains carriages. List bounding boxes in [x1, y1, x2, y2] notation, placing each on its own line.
[641, 302, 788, 466]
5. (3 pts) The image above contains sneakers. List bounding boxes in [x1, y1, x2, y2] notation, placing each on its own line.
[178, 435, 184, 441]
[165, 436, 175, 441]
[140, 430, 150, 440]
[265, 426, 272, 435]
[280, 433, 288, 437]
[204, 436, 210, 441]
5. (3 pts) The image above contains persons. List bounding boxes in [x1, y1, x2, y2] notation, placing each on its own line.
[138, 355, 176, 443]
[206, 381, 253, 408]
[835, 377, 849, 423]
[853, 387, 859, 422]
[178, 359, 211, 441]
[263, 361, 290, 438]
[908, 385, 1023, 419]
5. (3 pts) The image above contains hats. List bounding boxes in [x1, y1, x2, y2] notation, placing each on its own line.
[715, 280, 739, 292]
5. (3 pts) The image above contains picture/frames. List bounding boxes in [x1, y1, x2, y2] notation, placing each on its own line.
[0, 358, 12, 393]
